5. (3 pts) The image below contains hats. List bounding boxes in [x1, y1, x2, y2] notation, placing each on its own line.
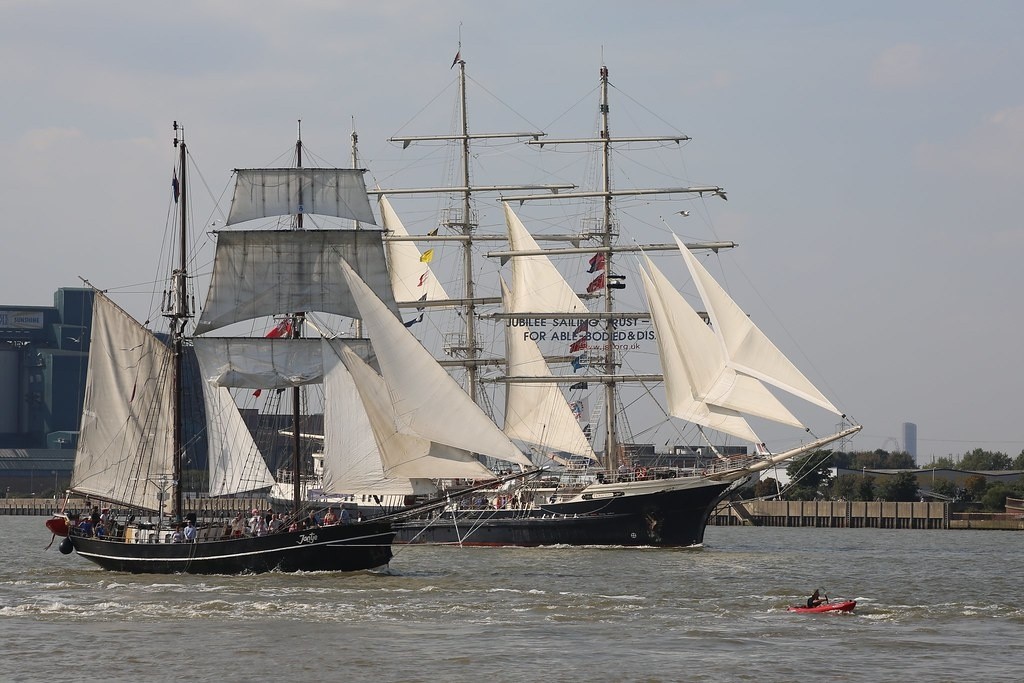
[811, 588, 819, 596]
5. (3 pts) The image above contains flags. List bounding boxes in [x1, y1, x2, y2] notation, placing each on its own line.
[253, 389, 261, 397]
[586, 252, 606, 293]
[569, 318, 588, 391]
[265, 318, 292, 338]
[402, 227, 439, 327]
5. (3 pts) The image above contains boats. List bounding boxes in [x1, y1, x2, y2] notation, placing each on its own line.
[786, 600, 857, 616]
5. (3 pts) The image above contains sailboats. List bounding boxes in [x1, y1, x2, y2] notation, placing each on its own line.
[43, 119, 549, 578]
[267, 22, 864, 553]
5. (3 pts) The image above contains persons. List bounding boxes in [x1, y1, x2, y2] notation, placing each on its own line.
[170, 520, 196, 544]
[807, 589, 825, 609]
[230, 503, 350, 537]
[460, 494, 535, 510]
[499, 469, 512, 475]
[78, 505, 119, 540]
